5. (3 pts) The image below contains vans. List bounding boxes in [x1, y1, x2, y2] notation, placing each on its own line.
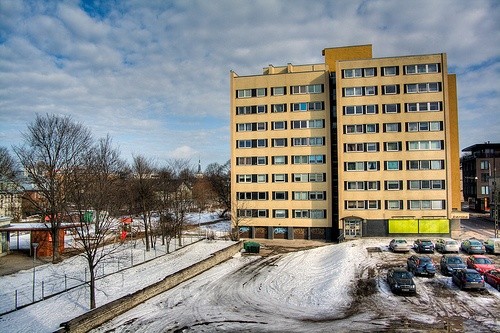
[485, 237, 500, 254]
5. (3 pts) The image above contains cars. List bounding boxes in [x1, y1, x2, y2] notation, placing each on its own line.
[387, 267, 416, 295]
[451, 268, 485, 290]
[389, 238, 409, 253]
[484, 268, 500, 291]
[413, 238, 434, 253]
[461, 239, 487, 254]
[435, 238, 460, 254]
[440, 254, 468, 276]
[467, 255, 496, 275]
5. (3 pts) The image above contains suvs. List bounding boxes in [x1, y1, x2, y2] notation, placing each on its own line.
[407, 254, 435, 276]
